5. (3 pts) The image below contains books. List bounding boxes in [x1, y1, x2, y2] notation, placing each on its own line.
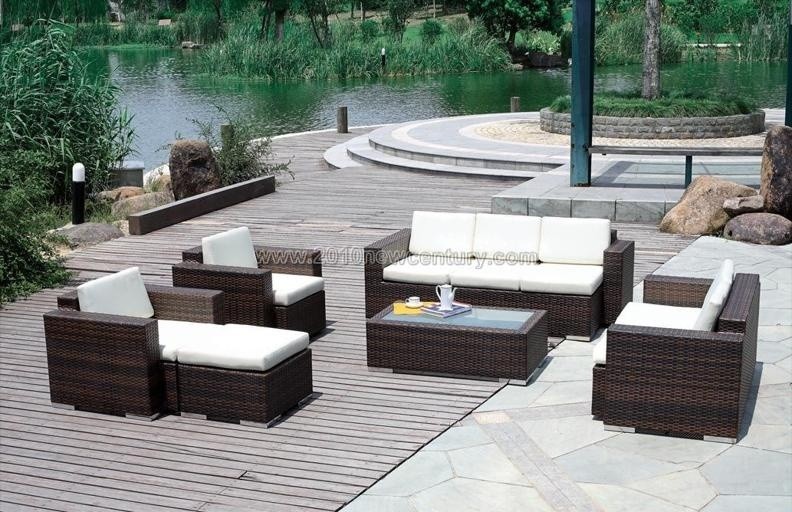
[421, 302, 472, 318]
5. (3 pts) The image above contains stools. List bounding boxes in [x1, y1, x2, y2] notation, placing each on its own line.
[173, 323, 319, 430]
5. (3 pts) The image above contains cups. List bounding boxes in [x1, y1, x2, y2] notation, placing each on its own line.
[404, 296, 421, 305]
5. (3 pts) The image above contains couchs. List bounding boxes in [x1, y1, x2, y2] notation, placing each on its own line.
[585, 259, 762, 445]
[171, 225, 330, 342]
[359, 209, 636, 343]
[40, 266, 227, 420]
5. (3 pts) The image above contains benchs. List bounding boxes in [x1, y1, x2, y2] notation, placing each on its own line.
[585, 141, 764, 192]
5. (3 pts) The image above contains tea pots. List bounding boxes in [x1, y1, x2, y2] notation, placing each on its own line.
[436, 284, 457, 311]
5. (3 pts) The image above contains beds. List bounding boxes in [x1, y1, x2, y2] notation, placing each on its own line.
[363, 296, 550, 387]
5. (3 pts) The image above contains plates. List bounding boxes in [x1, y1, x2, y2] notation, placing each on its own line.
[404, 304, 424, 308]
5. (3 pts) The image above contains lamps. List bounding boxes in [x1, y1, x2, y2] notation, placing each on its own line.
[69, 161, 87, 225]
[381, 47, 387, 67]
